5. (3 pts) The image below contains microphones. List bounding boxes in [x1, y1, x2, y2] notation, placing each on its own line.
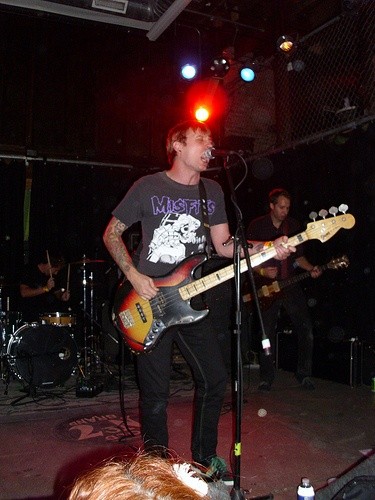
[59, 348, 66, 355]
[204, 147, 236, 159]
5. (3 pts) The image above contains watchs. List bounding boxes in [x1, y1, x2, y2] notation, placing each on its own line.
[44, 287, 48, 292]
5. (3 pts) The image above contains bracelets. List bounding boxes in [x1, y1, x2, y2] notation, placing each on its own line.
[264, 241, 273, 250]
[260, 268, 263, 276]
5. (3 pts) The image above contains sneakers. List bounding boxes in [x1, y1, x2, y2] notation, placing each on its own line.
[193, 454, 235, 486]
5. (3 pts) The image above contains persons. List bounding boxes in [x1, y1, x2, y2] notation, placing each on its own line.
[241, 188, 323, 392]
[102, 121, 296, 487]
[17, 252, 89, 394]
[62, 454, 215, 500]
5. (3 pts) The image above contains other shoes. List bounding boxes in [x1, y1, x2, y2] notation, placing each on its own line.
[257, 380, 271, 392]
[297, 373, 315, 390]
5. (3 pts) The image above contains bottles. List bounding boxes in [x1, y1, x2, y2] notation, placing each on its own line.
[297, 478, 315, 500]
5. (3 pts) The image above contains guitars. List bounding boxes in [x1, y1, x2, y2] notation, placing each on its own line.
[111, 203, 356, 357]
[240, 253, 350, 316]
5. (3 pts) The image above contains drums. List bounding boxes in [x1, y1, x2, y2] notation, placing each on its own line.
[0, 311, 22, 356]
[38, 312, 77, 326]
[6, 321, 77, 389]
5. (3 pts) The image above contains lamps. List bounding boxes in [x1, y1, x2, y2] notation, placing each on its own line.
[239, 61, 256, 83]
[278, 36, 293, 52]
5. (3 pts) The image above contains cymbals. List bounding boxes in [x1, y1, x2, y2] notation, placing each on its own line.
[73, 259, 104, 263]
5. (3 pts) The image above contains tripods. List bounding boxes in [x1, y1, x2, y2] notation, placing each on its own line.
[71, 262, 120, 381]
[4, 349, 64, 405]
